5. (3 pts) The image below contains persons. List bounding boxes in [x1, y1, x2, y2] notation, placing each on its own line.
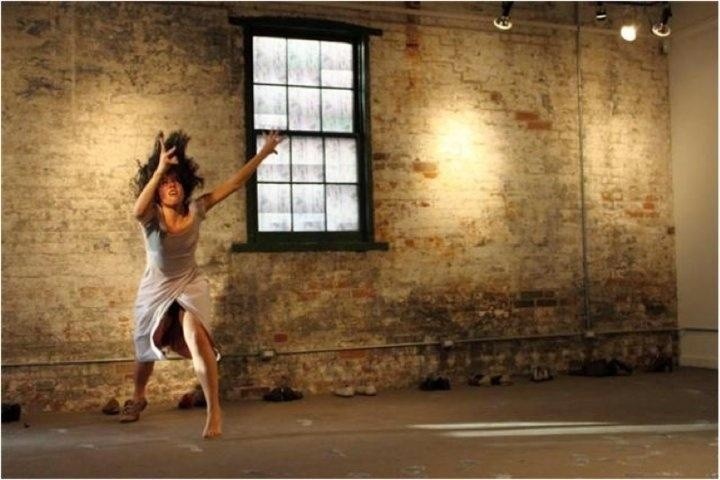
[119, 126, 287, 439]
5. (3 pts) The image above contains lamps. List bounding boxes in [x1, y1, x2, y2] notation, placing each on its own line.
[651, 3, 673, 39]
[493, 1, 514, 30]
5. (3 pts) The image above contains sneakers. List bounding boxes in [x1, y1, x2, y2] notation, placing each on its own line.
[120, 396, 148, 422]
